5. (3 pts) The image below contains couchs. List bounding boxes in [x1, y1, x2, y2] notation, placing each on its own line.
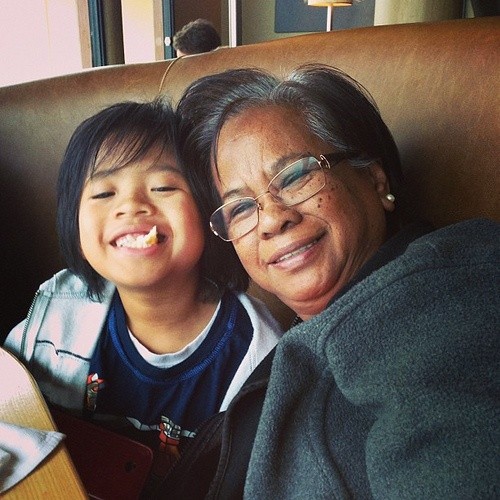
[0, 16, 500, 331]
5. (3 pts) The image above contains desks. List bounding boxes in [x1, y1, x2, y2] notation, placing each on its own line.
[0, 347, 90, 500]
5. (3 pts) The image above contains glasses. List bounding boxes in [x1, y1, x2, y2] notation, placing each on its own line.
[209, 150, 365, 241]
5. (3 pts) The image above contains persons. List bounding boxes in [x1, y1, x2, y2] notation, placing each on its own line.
[3, 102, 283, 500]
[165, 17, 235, 62]
[172, 65, 500, 500]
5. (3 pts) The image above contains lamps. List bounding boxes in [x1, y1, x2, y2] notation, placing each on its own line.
[307, 0, 353, 32]
